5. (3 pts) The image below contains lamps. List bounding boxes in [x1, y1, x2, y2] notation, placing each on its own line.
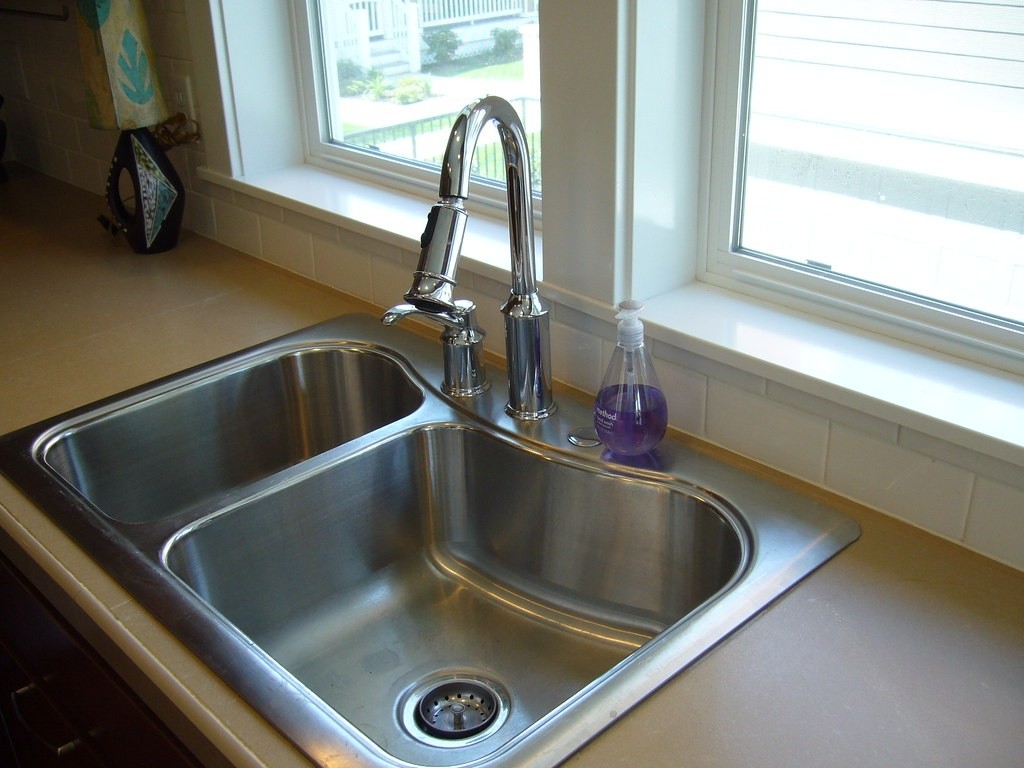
[75, 0, 202, 256]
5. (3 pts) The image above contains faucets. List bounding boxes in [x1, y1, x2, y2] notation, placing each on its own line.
[403, 95, 559, 422]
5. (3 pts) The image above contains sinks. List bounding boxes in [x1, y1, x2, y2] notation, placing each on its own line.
[31, 339, 425, 530]
[156, 418, 759, 768]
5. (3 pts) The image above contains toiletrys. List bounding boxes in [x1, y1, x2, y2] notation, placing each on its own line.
[594, 299, 668, 462]
